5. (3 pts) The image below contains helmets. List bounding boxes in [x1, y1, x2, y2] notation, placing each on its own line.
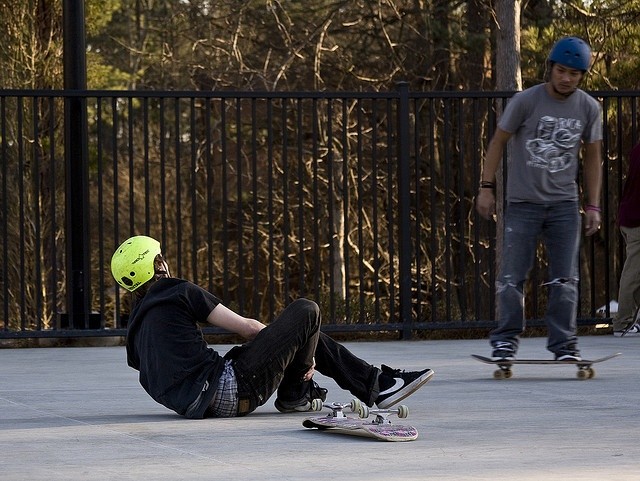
[548, 36, 591, 71]
[111, 235, 162, 291]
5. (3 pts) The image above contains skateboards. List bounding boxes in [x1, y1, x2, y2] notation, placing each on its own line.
[621, 306, 640, 337]
[470, 353, 622, 381]
[302, 399, 418, 441]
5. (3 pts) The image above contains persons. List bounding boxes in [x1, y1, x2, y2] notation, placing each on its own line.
[474, 35, 606, 361]
[110, 234, 432, 421]
[612, 138, 640, 337]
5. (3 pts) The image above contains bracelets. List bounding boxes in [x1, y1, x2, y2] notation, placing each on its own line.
[584, 204, 601, 213]
[480, 180, 495, 189]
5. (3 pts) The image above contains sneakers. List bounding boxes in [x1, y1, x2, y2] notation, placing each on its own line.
[375, 363, 434, 408]
[613, 322, 640, 337]
[491, 341, 516, 360]
[274, 381, 328, 412]
[555, 342, 582, 360]
